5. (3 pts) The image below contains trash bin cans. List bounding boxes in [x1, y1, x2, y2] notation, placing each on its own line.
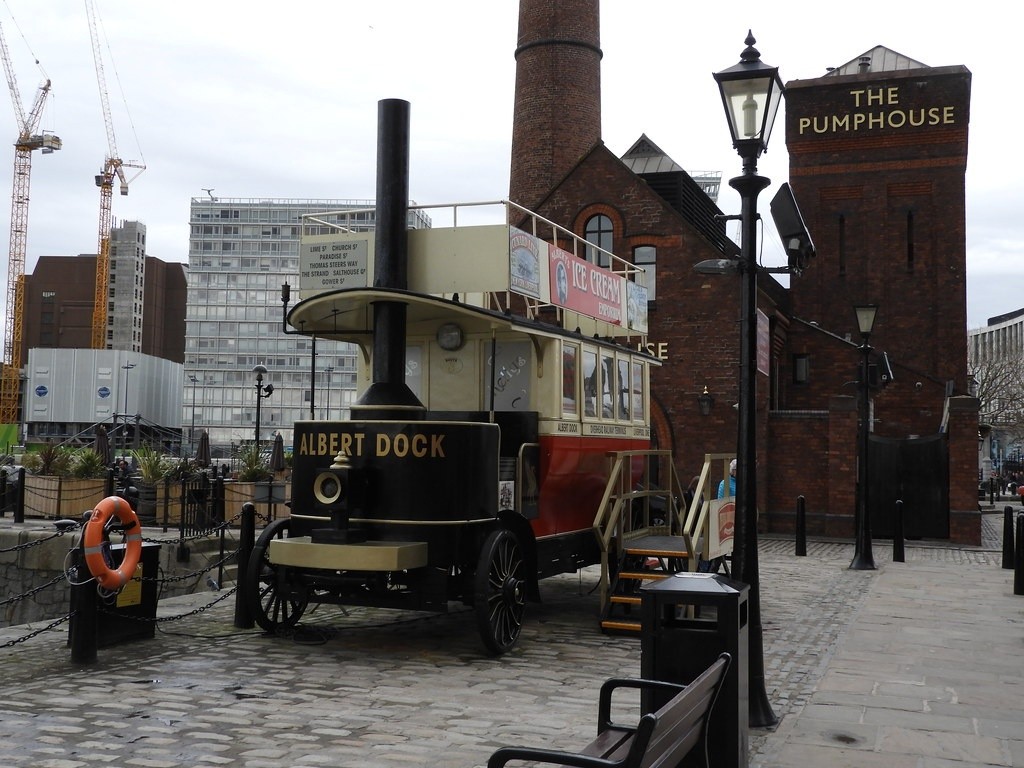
[641, 570, 751, 768]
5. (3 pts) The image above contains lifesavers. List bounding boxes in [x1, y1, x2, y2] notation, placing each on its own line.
[84, 493, 143, 591]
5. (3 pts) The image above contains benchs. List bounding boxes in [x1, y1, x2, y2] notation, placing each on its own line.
[484, 651, 731, 768]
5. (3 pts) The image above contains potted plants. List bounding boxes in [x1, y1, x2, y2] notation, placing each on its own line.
[20, 440, 108, 520]
[129, 439, 286, 527]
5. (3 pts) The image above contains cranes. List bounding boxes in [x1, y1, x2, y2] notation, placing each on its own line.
[0, 0, 63, 424]
[85, 0, 148, 350]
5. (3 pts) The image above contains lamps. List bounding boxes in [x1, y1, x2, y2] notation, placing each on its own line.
[979, 424, 994, 452]
[792, 352, 810, 385]
[697, 385, 715, 416]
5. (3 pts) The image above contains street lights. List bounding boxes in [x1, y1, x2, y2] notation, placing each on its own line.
[189, 374, 199, 454]
[122, 359, 137, 461]
[252, 361, 274, 461]
[848, 301, 878, 571]
[712, 31, 787, 731]
